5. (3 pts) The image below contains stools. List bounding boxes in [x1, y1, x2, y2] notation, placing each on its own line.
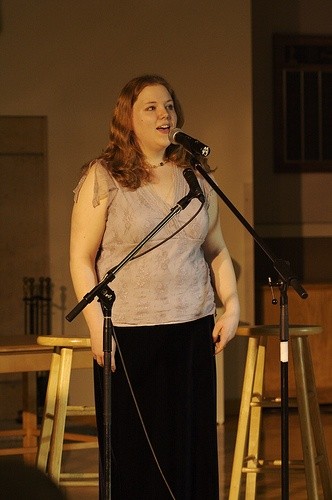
[226, 322, 332, 500]
[36, 335, 100, 492]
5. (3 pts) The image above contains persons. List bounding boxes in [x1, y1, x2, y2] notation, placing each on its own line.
[68, 73, 241, 500]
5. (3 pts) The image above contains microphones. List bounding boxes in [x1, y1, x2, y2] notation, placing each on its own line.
[183, 168, 205, 203]
[168, 128, 210, 157]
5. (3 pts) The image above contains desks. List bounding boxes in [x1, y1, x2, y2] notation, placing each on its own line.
[0, 344, 94, 471]
